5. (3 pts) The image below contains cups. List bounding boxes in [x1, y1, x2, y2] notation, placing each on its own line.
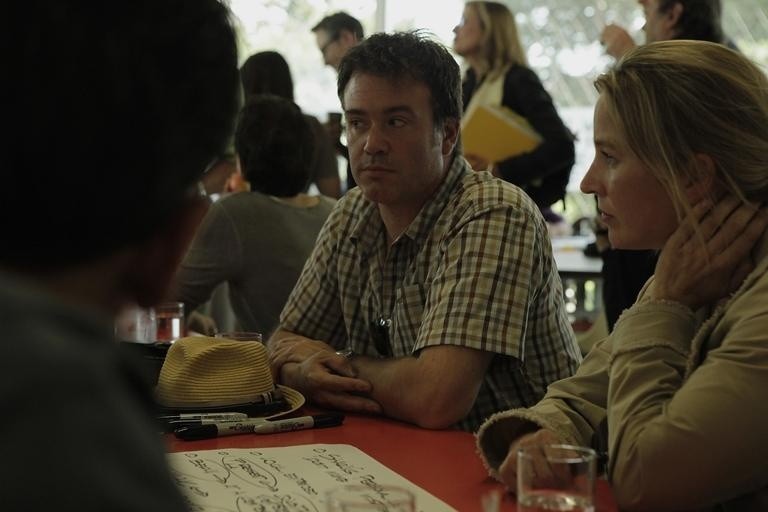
[515, 444, 596, 512]
[149, 302, 186, 344]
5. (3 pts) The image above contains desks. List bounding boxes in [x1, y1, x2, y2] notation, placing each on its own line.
[157, 402, 619, 512]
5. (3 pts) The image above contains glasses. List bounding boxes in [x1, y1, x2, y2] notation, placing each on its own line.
[318, 32, 340, 52]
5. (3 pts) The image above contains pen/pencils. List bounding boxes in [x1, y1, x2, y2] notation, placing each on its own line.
[156, 411, 345, 441]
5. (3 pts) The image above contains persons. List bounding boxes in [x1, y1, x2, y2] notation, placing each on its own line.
[158, 11, 364, 343]
[1, 1, 244, 512]
[266, 28, 607, 472]
[583, 1, 740, 336]
[475, 40, 767, 512]
[453, 2, 579, 211]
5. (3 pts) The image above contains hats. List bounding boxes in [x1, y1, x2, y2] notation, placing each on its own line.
[152, 335, 306, 424]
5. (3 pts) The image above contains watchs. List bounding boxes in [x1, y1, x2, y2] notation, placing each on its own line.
[335, 348, 361, 361]
